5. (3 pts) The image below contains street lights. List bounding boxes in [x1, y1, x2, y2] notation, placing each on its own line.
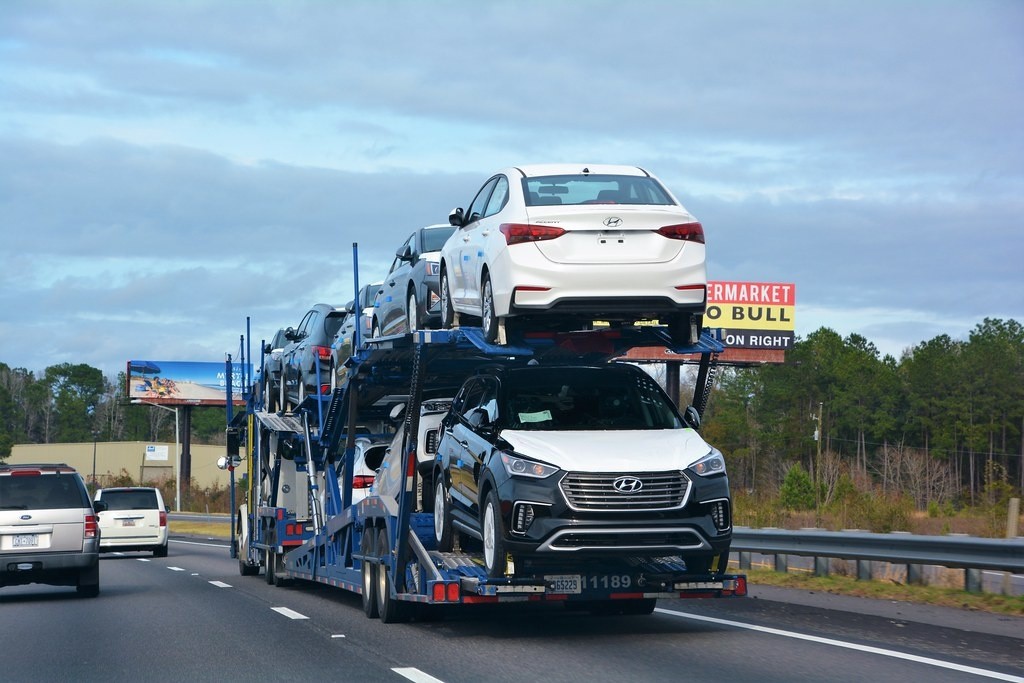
[129, 398, 181, 513]
[91, 431, 101, 496]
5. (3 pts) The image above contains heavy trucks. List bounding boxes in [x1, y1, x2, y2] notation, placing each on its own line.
[217, 242, 752, 625]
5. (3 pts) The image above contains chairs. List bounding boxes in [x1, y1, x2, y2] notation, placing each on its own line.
[586, 396, 630, 425]
[47, 487, 73, 506]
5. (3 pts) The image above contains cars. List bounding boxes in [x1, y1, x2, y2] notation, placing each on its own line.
[327, 281, 388, 396]
[437, 163, 708, 346]
[94, 485, 171, 557]
[262, 327, 298, 413]
[430, 364, 733, 577]
[371, 222, 460, 340]
[367, 395, 464, 518]
[319, 434, 398, 517]
[279, 303, 351, 413]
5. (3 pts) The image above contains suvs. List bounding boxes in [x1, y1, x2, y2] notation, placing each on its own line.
[0, 462, 109, 596]
